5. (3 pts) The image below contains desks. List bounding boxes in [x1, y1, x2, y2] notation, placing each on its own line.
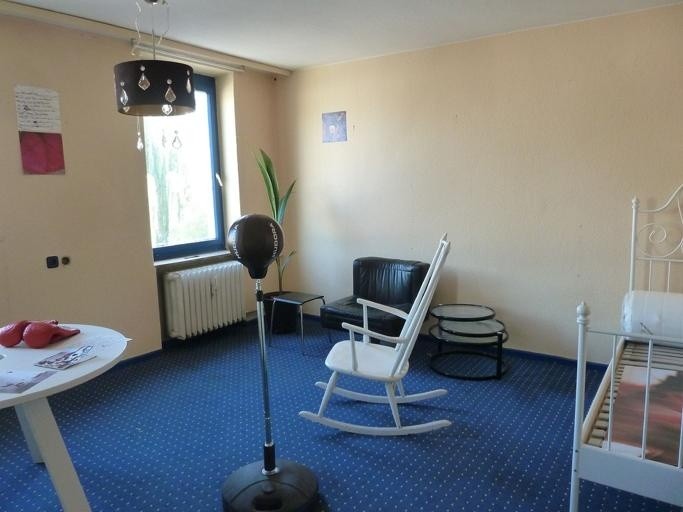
[0, 320, 127, 511]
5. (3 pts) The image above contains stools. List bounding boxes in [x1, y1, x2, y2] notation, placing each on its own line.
[268, 291, 335, 356]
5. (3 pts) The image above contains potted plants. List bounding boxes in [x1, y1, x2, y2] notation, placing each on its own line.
[250, 146, 300, 335]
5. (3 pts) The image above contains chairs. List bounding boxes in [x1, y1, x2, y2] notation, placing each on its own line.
[298, 232, 453, 438]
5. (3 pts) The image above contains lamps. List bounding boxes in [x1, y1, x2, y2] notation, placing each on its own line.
[112, 0, 196, 152]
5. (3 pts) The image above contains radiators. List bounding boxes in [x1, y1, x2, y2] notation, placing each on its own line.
[161, 259, 249, 343]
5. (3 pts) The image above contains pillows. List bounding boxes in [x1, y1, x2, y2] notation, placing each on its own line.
[618, 290, 683, 347]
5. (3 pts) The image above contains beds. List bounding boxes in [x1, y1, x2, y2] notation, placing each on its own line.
[565, 184, 683, 512]
